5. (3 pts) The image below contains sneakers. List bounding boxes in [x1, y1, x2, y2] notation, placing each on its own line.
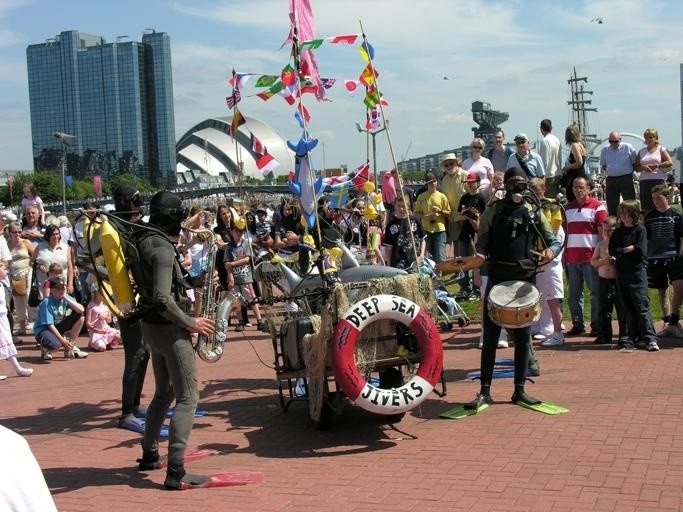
[257, 322, 264, 330]
[478, 322, 682, 353]
[12, 327, 25, 342]
[40, 347, 52, 361]
[63, 345, 88, 359]
[235, 318, 244, 330]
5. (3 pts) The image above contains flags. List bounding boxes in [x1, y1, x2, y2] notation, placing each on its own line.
[226, 0, 395, 118]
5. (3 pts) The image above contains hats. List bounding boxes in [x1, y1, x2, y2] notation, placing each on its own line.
[422, 171, 436, 184]
[48, 276, 67, 288]
[462, 173, 481, 184]
[513, 132, 527, 141]
[438, 152, 460, 164]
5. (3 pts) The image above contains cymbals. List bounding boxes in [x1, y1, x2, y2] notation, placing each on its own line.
[436, 255, 484, 272]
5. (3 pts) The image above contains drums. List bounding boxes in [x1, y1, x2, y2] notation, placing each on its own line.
[487, 281, 542, 328]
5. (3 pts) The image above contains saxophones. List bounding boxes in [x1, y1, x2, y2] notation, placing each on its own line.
[181, 226, 245, 363]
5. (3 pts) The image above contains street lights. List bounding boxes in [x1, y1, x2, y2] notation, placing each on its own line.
[54, 131, 75, 216]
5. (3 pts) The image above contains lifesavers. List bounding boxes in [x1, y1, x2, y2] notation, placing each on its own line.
[332, 294, 444, 415]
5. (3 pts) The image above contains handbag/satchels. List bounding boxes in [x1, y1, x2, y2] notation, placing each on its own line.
[10, 275, 28, 296]
[27, 285, 44, 308]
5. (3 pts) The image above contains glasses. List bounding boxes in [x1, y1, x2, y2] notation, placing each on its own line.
[644, 136, 654, 140]
[472, 146, 482, 149]
[608, 139, 620, 144]
[443, 163, 454, 167]
[11, 230, 22, 234]
[162, 206, 188, 219]
[517, 141, 525, 145]
[120, 193, 143, 209]
[505, 180, 527, 192]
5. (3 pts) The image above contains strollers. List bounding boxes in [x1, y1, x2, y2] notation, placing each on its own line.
[412, 256, 473, 338]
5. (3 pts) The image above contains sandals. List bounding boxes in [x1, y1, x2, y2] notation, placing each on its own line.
[16, 368, 34, 378]
